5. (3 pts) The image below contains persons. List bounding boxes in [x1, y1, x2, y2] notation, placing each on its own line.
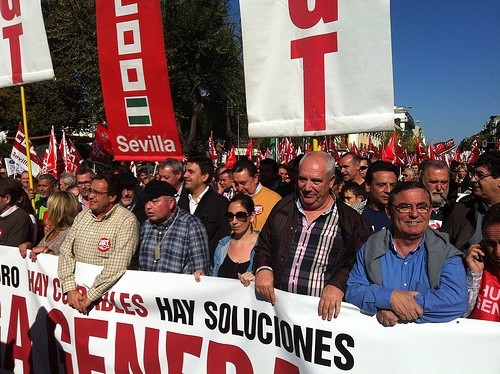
[0, 168, 39, 247]
[344, 150, 500, 328]
[193, 153, 304, 288]
[29, 158, 231, 315]
[252, 151, 373, 321]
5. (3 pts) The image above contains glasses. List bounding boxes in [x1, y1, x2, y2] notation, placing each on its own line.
[391, 204, 432, 214]
[76, 181, 91, 186]
[224, 210, 255, 222]
[360, 166, 369, 171]
[469, 171, 492, 179]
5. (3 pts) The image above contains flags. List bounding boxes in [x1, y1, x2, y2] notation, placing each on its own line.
[206, 130, 479, 175]
[59, 128, 86, 177]
[10, 122, 43, 180]
[86, 124, 134, 174]
[41, 125, 65, 181]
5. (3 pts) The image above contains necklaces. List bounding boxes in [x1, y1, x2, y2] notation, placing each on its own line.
[152, 207, 179, 260]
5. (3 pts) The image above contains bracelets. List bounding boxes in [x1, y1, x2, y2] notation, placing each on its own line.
[41, 246, 48, 253]
[254, 265, 273, 273]
[45, 249, 51, 254]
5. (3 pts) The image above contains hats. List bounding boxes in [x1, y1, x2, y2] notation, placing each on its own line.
[136, 179, 178, 199]
[115, 173, 139, 189]
